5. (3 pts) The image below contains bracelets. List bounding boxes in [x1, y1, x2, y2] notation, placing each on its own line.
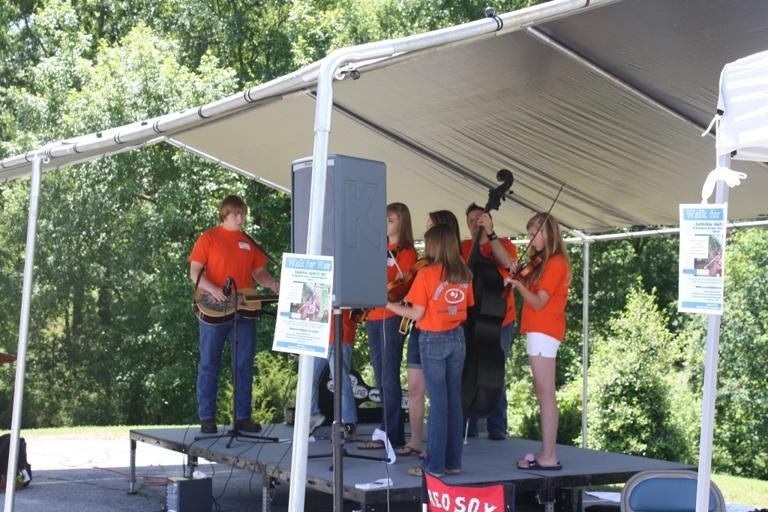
[488, 231, 497, 240]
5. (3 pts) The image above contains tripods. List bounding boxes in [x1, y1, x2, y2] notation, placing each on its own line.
[302, 311, 391, 473]
[194, 341, 280, 448]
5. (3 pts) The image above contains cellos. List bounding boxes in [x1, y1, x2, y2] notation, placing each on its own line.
[463, 169, 513, 446]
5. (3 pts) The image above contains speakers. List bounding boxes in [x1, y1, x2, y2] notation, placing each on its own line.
[290, 155, 389, 308]
[166, 474, 213, 512]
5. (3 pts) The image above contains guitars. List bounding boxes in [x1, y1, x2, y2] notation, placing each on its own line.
[193, 288, 280, 325]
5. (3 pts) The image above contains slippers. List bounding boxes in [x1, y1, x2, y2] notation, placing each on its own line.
[393, 446, 430, 460]
[517, 453, 562, 470]
[359, 441, 384, 448]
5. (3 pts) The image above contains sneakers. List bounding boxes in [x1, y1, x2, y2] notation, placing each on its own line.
[235, 419, 261, 432]
[464, 431, 479, 438]
[408, 464, 462, 478]
[488, 431, 505, 440]
[201, 418, 218, 433]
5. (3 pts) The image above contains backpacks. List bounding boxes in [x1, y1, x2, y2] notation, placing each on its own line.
[0, 434, 32, 491]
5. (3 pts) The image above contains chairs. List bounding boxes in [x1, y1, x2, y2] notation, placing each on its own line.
[420, 468, 515, 512]
[619, 469, 728, 511]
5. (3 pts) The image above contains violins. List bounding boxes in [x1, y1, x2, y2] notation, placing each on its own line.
[349, 258, 430, 324]
[502, 255, 544, 298]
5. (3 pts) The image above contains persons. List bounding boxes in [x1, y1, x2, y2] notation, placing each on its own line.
[461, 201, 519, 439]
[186, 195, 281, 432]
[385, 224, 475, 478]
[396, 210, 463, 457]
[357, 202, 418, 448]
[306, 308, 358, 441]
[504, 213, 572, 469]
[300, 284, 320, 320]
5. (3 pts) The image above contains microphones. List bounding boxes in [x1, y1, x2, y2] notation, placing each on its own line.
[221, 277, 233, 296]
